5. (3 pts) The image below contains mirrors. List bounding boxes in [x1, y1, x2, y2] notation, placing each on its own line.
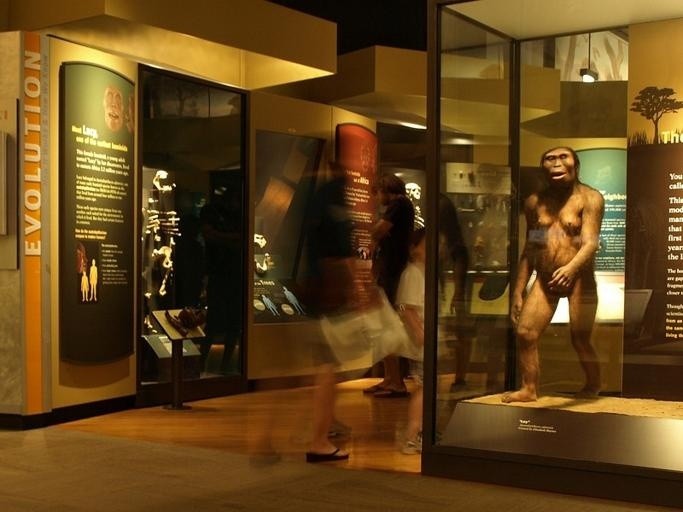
[129, 59, 251, 412]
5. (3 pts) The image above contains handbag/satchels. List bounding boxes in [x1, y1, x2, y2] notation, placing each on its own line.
[319, 287, 408, 383]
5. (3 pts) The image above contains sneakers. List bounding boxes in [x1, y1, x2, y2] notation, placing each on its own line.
[401, 430, 422, 455]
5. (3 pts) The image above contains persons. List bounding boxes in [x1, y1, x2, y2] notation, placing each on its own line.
[247, 164, 507, 464]
[104, 86, 124, 130]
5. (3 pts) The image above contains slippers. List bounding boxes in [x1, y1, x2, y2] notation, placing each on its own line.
[361, 383, 412, 399]
[306, 443, 351, 464]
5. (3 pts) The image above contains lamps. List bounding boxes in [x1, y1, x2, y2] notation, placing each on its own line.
[578, 33, 598, 84]
